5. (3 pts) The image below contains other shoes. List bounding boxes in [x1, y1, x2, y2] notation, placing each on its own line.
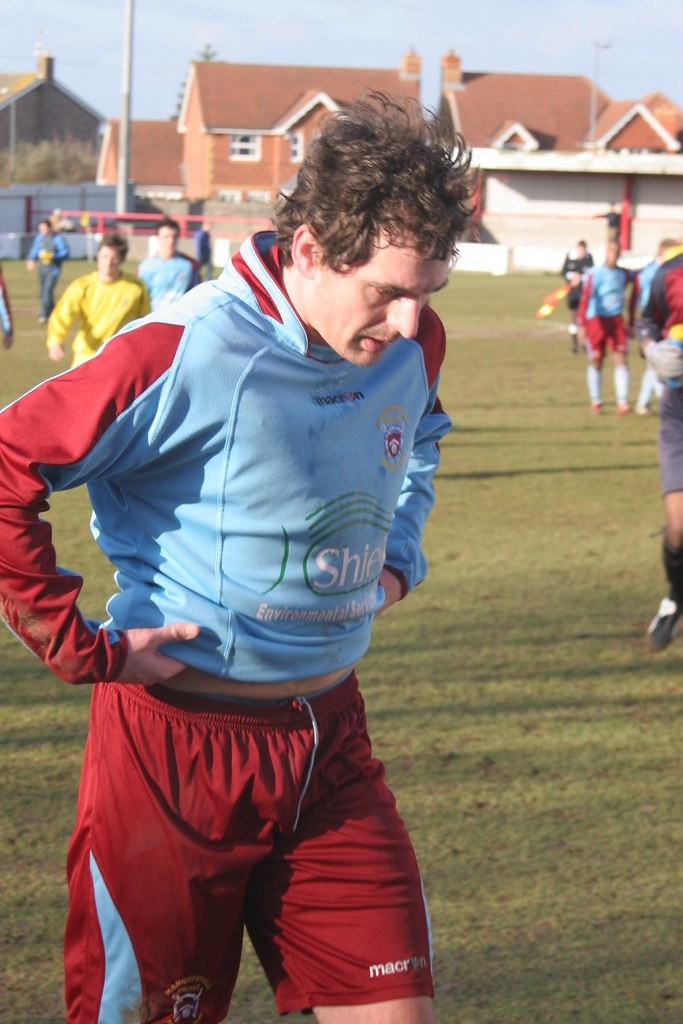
[634, 404, 651, 416]
[590, 401, 602, 414]
[616, 401, 630, 415]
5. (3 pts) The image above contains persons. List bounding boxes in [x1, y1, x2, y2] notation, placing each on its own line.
[47, 234, 150, 369]
[138, 220, 203, 314]
[638, 252, 682, 649]
[2, 276, 14, 351]
[194, 221, 215, 283]
[0, 88, 475, 1024]
[25, 221, 69, 323]
[562, 240, 682, 413]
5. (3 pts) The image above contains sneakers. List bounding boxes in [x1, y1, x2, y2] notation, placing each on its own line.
[645, 596, 683, 651]
